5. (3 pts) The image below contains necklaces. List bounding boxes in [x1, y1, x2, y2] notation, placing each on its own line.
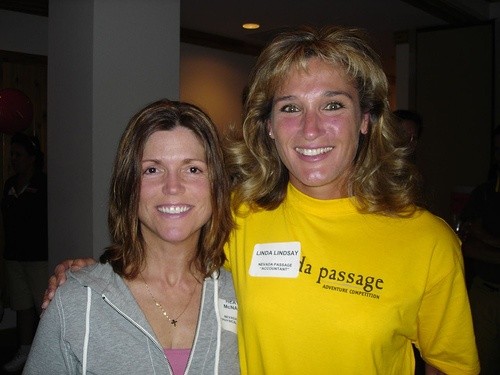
[138, 267, 202, 327]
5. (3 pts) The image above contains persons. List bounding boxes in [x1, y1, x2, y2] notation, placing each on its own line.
[0, 133, 47, 372]
[388, 110, 439, 215]
[22, 98, 242, 374]
[41, 26, 500, 375]
